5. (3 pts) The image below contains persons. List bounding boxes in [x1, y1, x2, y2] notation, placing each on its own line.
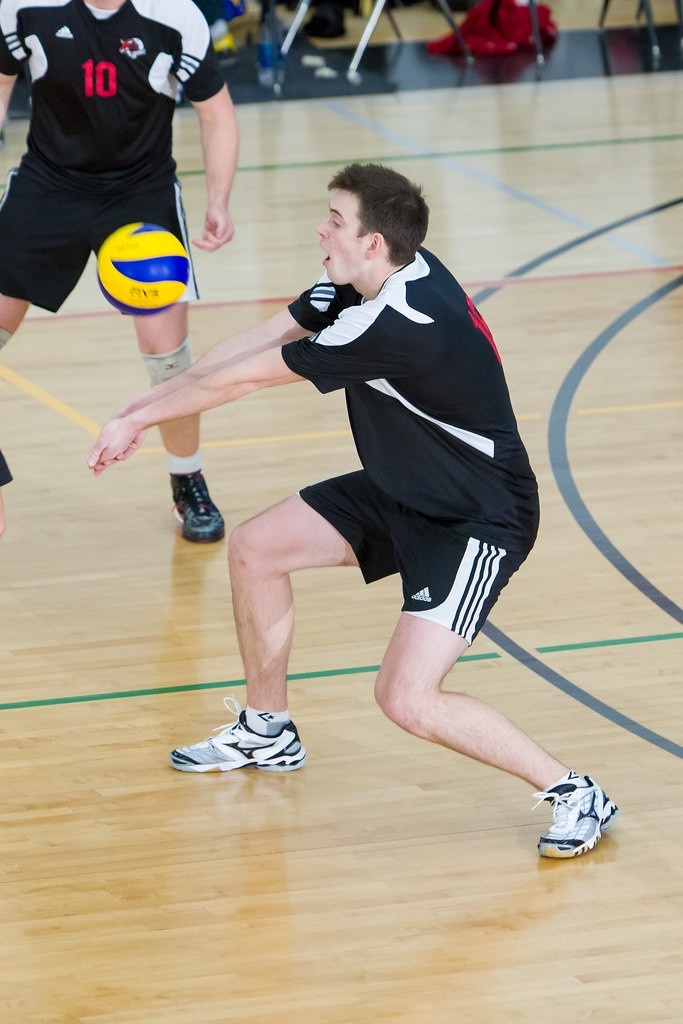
[87, 162, 622, 860]
[0, 0, 239, 545]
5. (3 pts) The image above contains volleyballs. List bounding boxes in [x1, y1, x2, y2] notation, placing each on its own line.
[95, 219, 192, 317]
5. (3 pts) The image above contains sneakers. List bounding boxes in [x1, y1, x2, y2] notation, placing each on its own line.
[169, 468, 224, 543]
[531, 775, 620, 858]
[169, 692, 307, 773]
